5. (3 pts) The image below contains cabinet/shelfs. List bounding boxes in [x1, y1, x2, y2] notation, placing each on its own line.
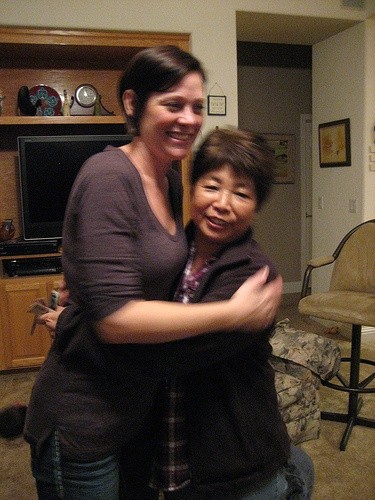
[0, 26, 195, 372]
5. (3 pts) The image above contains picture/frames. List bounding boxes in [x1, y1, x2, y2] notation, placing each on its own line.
[257, 131, 297, 185]
[318, 117, 351, 168]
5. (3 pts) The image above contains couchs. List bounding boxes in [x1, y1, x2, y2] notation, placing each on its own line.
[269, 316, 343, 447]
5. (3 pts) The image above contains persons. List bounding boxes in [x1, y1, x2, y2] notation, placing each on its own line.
[37, 128, 313, 500]
[23, 45, 284, 500]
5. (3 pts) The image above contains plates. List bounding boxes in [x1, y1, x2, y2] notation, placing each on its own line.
[29, 86, 62, 116]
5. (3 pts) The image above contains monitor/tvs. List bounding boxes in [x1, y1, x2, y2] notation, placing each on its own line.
[18, 133, 174, 242]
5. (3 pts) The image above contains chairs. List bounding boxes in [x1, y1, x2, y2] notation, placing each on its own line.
[298, 218, 375, 452]
